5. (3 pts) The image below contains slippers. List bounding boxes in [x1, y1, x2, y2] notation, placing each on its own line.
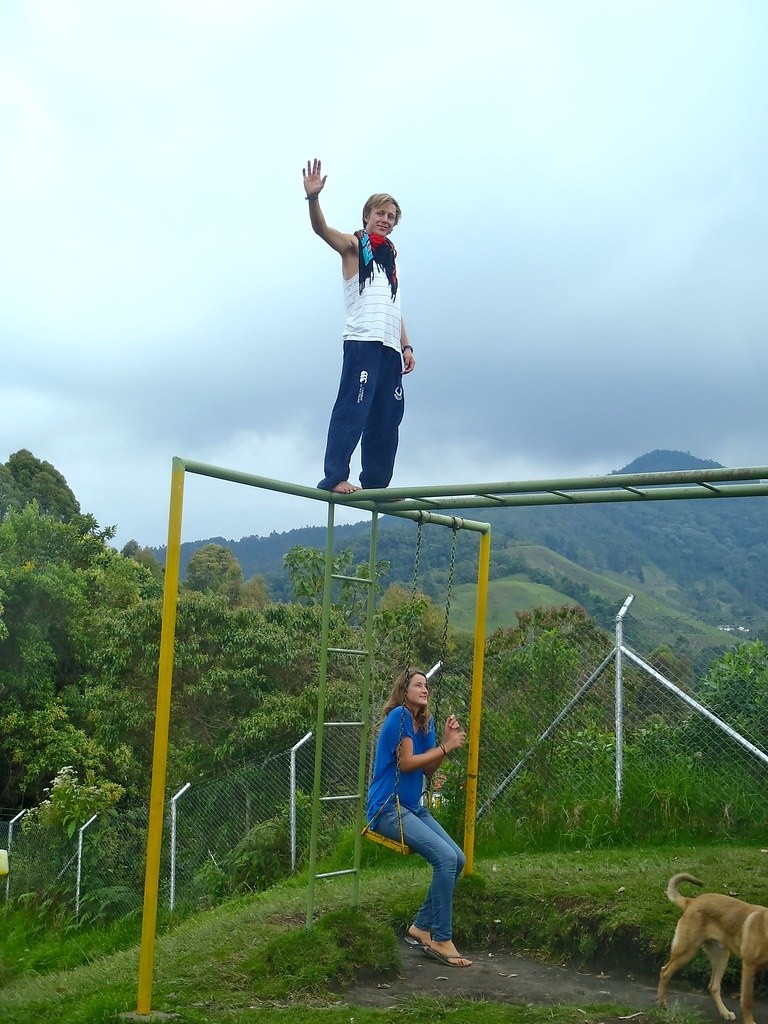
[424, 946, 473, 967]
[404, 936, 436, 959]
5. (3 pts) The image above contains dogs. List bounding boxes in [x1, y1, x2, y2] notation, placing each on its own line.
[656, 873, 768, 1024]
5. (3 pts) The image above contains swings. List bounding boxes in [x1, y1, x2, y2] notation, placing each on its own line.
[365, 521, 463, 854]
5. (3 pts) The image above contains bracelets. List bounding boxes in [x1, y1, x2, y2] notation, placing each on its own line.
[304, 196, 319, 201]
[439, 743, 447, 757]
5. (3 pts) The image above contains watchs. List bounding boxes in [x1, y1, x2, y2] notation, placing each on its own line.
[401, 345, 413, 354]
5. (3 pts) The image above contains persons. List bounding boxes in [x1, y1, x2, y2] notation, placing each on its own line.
[302, 158, 415, 503]
[367, 668, 473, 969]
